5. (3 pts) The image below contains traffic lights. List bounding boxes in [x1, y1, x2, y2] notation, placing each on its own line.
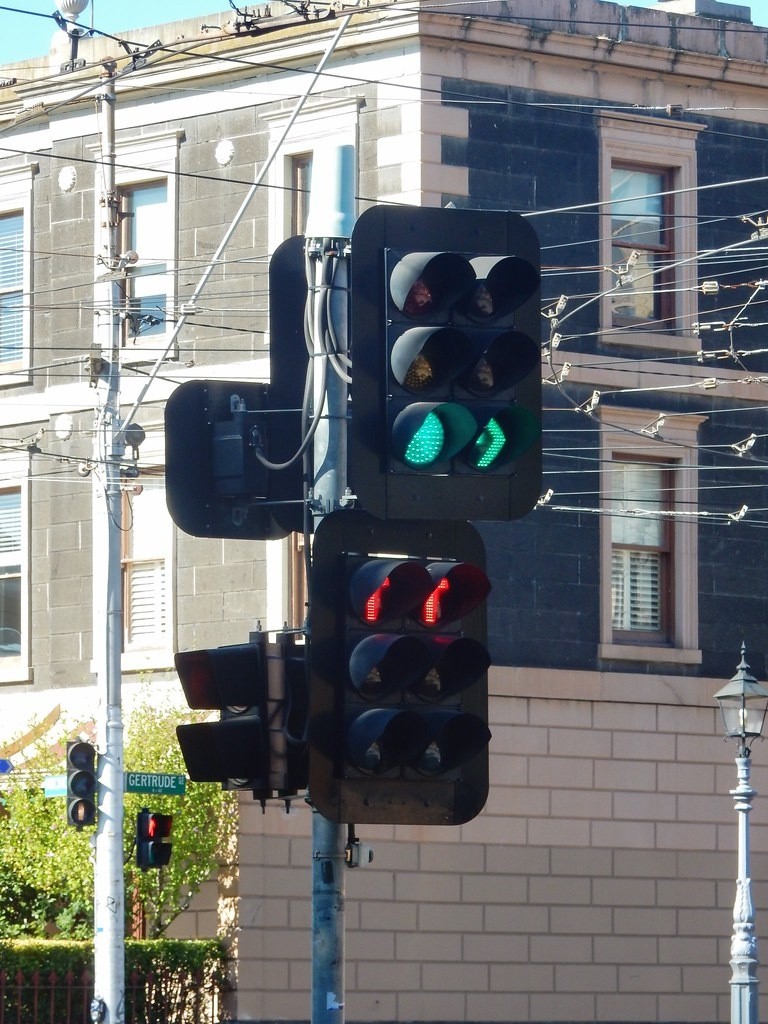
[66, 742, 96, 828]
[308, 512, 491, 826]
[170, 631, 269, 798]
[136, 809, 172, 872]
[272, 628, 309, 802]
[348, 205, 542, 521]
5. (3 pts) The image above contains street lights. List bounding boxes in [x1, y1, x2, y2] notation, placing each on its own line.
[711, 633, 768, 1024]
